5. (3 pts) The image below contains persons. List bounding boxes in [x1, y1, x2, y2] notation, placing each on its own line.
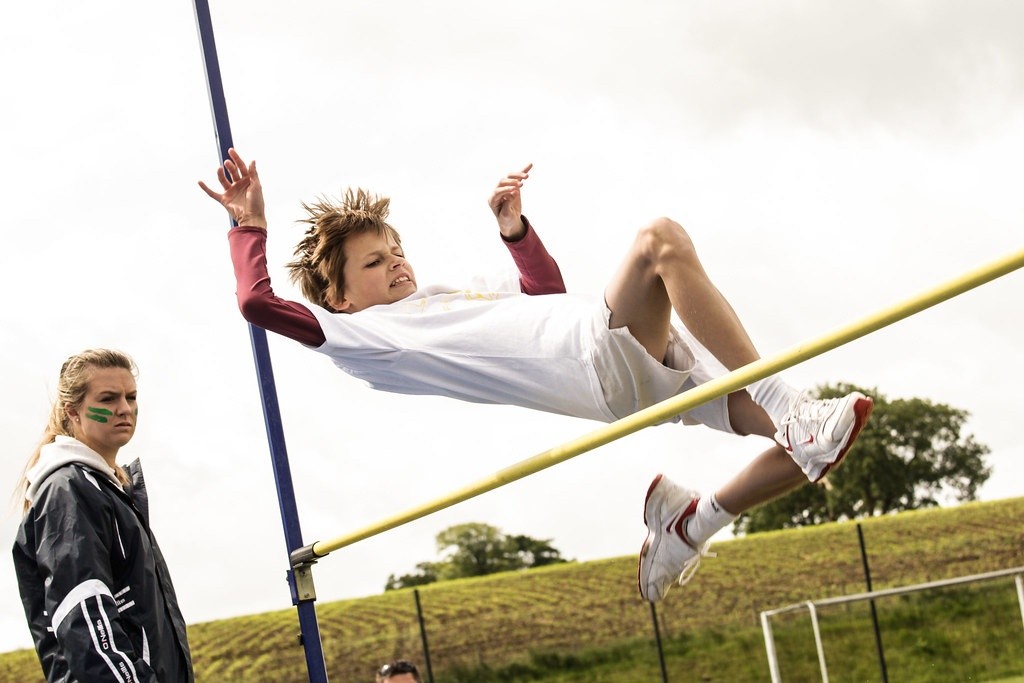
[376, 659, 422, 683]
[196, 146, 875, 602]
[12, 347, 196, 683]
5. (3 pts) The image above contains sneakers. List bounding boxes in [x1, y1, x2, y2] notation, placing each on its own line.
[774, 387, 874, 484]
[639, 473, 717, 601]
